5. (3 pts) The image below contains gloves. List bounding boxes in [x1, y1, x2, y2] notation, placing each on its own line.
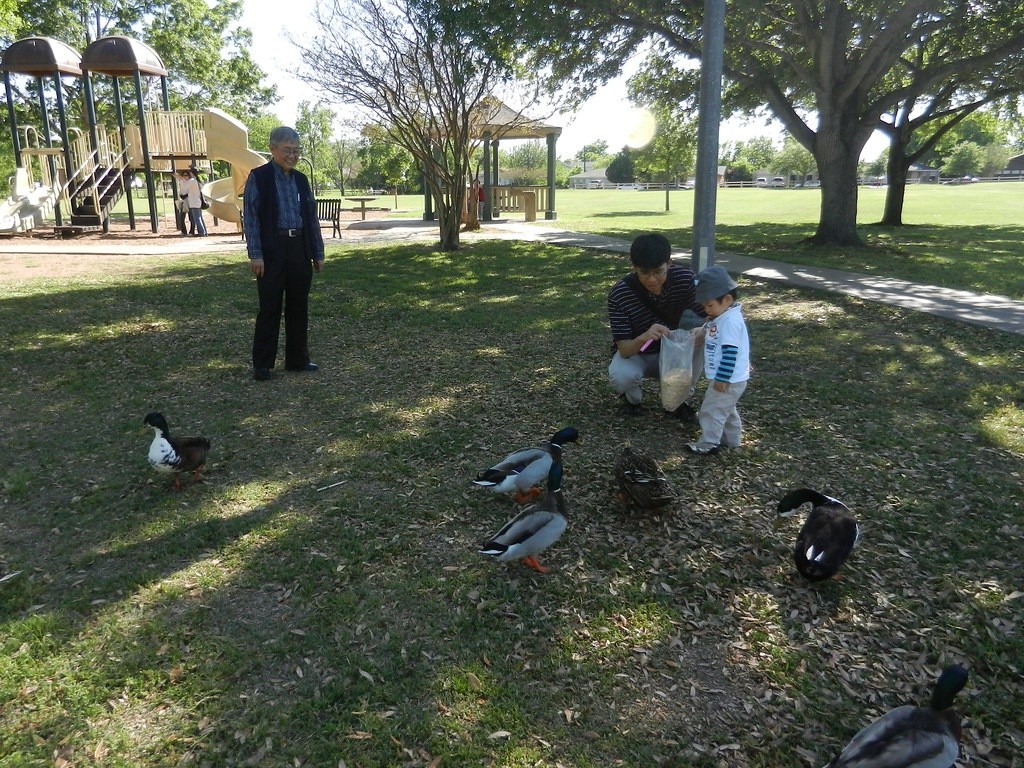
[180, 194, 188, 199]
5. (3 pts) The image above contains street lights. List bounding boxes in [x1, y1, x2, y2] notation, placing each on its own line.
[298, 156, 313, 196]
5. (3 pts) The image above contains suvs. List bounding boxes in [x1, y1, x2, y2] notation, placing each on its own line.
[756, 178, 768, 188]
[795, 180, 820, 188]
[772, 177, 786, 187]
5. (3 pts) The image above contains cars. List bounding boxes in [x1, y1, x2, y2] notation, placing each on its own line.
[943, 178, 964, 184]
[616, 184, 644, 191]
[587, 180, 603, 189]
[669, 181, 695, 191]
[964, 175, 981, 183]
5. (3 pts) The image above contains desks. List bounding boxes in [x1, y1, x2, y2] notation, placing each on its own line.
[345, 197, 380, 220]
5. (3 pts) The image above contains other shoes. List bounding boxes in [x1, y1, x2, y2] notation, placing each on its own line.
[684, 442, 718, 455]
[194, 234, 205, 236]
[187, 233, 194, 237]
[180, 233, 186, 236]
[305, 362, 319, 370]
[253, 366, 270, 380]
[626, 385, 642, 405]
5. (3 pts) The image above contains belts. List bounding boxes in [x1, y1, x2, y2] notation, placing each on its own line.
[276, 228, 303, 237]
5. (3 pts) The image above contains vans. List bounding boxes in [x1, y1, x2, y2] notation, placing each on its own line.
[130, 177, 143, 189]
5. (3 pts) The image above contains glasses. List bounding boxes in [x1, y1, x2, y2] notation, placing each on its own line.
[275, 145, 300, 156]
[635, 263, 668, 281]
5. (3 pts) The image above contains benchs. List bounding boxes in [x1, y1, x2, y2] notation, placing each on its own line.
[315, 199, 342, 239]
[381, 208, 391, 212]
[352, 207, 380, 210]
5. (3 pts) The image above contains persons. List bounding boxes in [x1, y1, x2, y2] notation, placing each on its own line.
[467, 179, 484, 220]
[609, 234, 709, 425]
[684, 267, 750, 455]
[243, 127, 325, 381]
[172, 167, 204, 237]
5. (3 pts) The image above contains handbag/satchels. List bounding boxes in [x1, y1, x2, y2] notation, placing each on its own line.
[200, 198, 209, 209]
[659, 330, 696, 412]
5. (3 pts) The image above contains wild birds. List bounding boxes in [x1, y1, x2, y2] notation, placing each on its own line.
[819, 663, 969, 768]
[614, 445, 678, 521]
[478, 460, 571, 574]
[771, 486, 861, 583]
[143, 412, 208, 495]
[473, 426, 580, 507]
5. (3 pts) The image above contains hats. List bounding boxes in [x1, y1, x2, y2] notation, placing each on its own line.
[689, 262, 739, 304]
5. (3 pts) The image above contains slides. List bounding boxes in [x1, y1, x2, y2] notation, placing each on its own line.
[200, 106, 268, 222]
[0, 167, 66, 233]
